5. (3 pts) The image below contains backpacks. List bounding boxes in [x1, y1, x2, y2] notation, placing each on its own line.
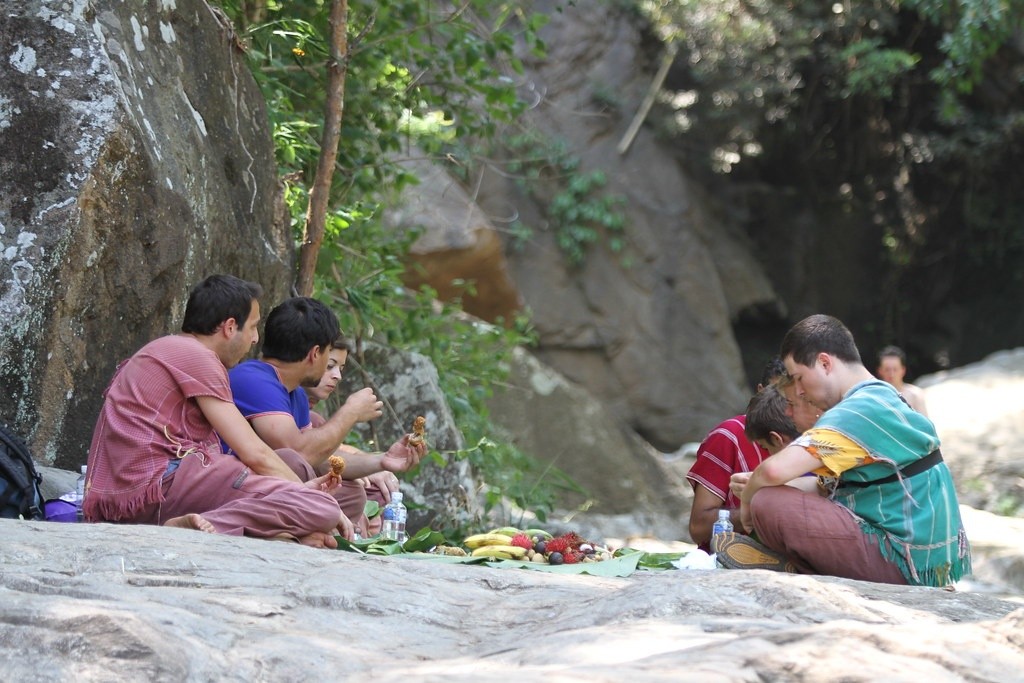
[0, 423, 47, 521]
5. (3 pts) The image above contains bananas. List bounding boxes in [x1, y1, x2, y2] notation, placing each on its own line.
[464, 527, 553, 560]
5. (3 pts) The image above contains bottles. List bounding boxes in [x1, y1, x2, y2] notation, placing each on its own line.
[712, 509, 735, 537]
[76, 465, 87, 524]
[381, 492, 407, 551]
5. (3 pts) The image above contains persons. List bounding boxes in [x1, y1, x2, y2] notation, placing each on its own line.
[876, 346, 927, 418]
[744, 385, 828, 477]
[709, 314, 973, 587]
[82, 274, 354, 549]
[213, 296, 426, 532]
[776, 375, 823, 433]
[300, 328, 400, 539]
[685, 354, 786, 556]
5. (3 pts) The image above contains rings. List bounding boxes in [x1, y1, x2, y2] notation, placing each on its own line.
[392, 480, 395, 481]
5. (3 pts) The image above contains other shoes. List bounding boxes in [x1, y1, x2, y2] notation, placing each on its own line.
[709, 531, 795, 572]
